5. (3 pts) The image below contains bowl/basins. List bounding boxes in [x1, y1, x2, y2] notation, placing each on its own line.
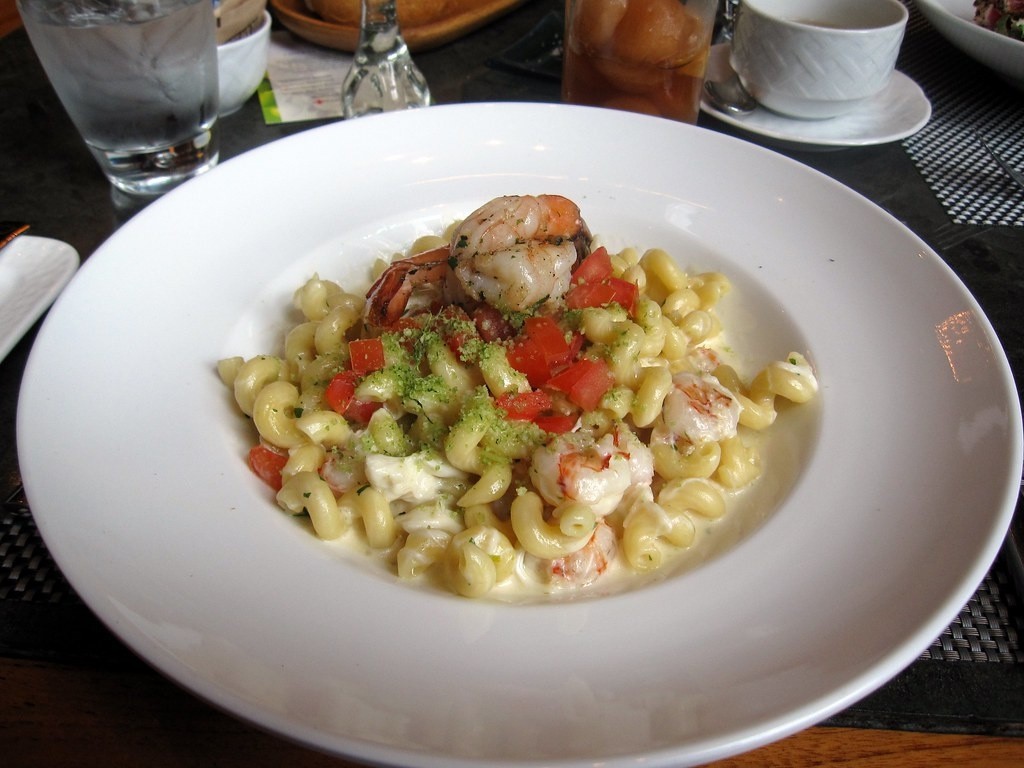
[211, 9, 273, 120]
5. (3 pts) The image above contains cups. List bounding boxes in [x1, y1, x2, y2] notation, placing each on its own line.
[728, 0, 908, 118]
[559, 0, 717, 126]
[13, 1, 221, 196]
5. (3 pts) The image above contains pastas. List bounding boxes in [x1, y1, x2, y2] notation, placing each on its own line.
[220, 196, 819, 599]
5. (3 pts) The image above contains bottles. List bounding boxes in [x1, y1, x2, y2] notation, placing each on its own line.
[339, 0, 431, 121]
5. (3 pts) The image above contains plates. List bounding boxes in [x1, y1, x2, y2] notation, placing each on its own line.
[913, 0, 1024, 79]
[10, 98, 1024, 767]
[267, 0, 516, 54]
[695, 42, 933, 154]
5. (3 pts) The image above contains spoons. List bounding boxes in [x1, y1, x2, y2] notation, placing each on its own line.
[702, 0, 758, 116]
[972, 129, 1024, 190]
